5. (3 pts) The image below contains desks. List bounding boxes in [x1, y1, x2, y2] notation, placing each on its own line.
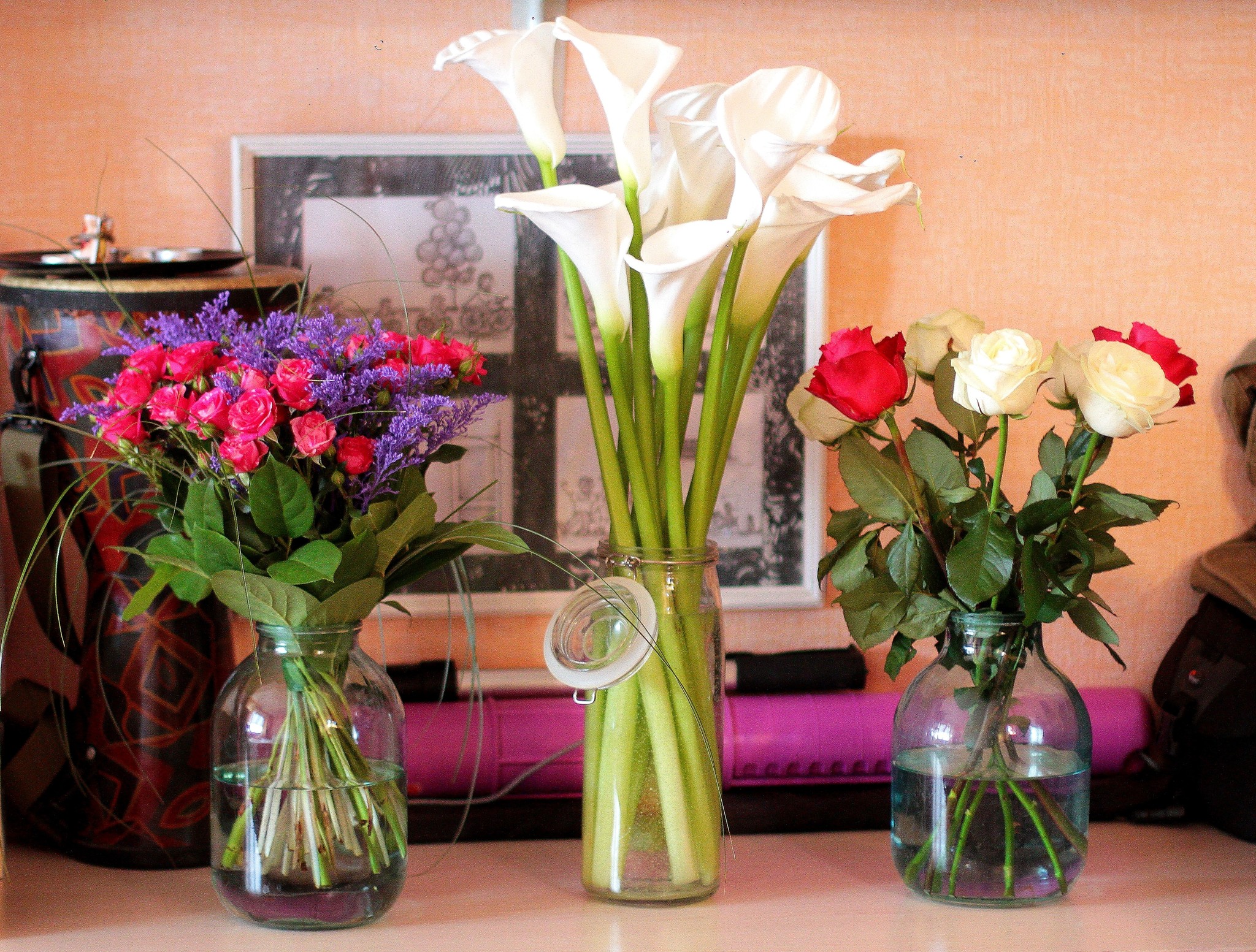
[0, 823, 1256, 952]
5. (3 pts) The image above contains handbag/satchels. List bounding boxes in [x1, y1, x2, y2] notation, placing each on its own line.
[1088, 595, 1256, 845]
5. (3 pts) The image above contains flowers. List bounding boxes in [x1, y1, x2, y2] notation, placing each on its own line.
[784, 309, 1198, 676]
[431, 15, 922, 535]
[0, 139, 509, 614]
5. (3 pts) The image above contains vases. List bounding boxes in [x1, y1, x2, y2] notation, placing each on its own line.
[208, 620, 409, 931]
[583, 538, 726, 904]
[891, 613, 1093, 908]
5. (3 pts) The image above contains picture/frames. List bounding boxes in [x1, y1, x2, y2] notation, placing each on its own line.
[232, 136, 827, 615]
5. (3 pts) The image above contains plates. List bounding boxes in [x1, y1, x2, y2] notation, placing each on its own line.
[0, 246, 251, 279]
